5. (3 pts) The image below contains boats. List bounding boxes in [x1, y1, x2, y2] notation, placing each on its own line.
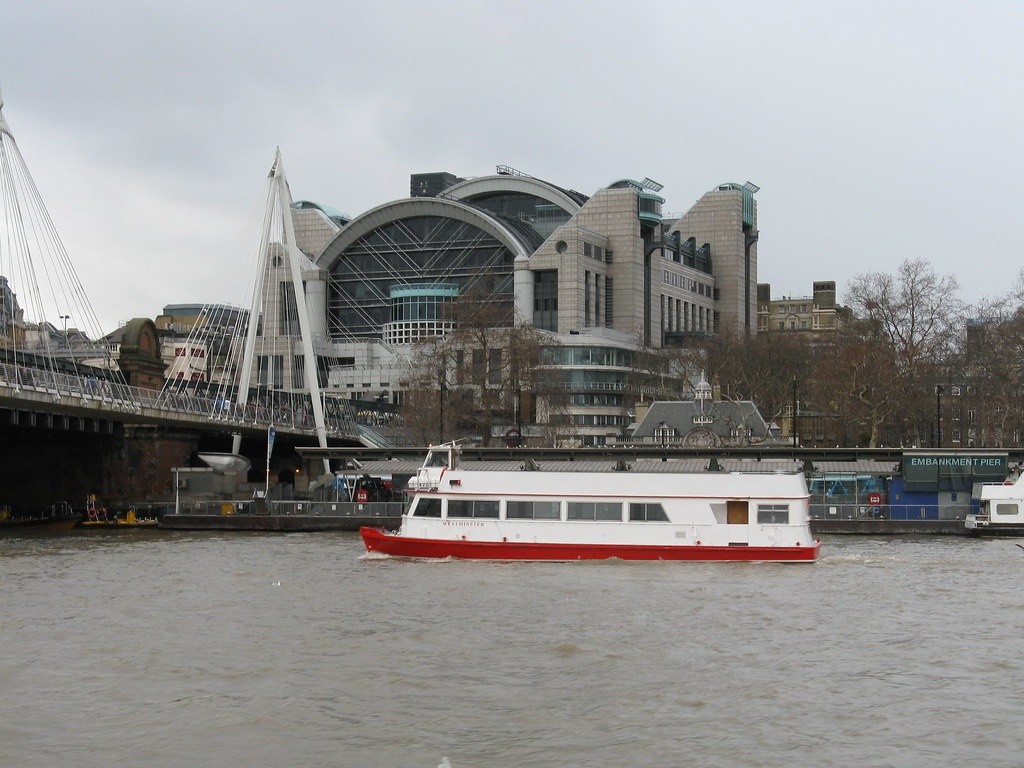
[75, 491, 159, 527]
[962, 464, 1024, 535]
[357, 433, 823, 565]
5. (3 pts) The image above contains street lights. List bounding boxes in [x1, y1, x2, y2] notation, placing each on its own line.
[792, 374, 798, 447]
[935, 384, 944, 446]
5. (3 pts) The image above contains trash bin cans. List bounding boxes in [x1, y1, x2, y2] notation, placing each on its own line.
[292, 490, 315, 514]
